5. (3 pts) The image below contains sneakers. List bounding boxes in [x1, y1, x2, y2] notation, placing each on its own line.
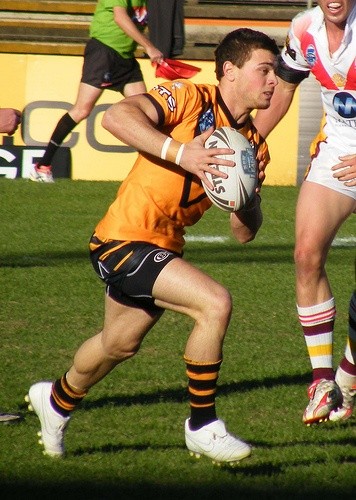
[24, 381, 72, 458]
[330, 363, 356, 423]
[303, 380, 342, 427]
[31, 160, 55, 184]
[184, 414, 253, 466]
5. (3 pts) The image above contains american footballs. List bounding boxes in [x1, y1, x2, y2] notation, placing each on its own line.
[201, 127, 257, 212]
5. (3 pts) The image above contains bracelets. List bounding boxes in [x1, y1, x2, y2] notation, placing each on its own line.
[160, 136, 184, 165]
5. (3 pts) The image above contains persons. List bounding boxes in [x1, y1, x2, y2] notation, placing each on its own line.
[29, 0, 164, 183]
[23, 27, 278, 462]
[252, 0, 356, 427]
